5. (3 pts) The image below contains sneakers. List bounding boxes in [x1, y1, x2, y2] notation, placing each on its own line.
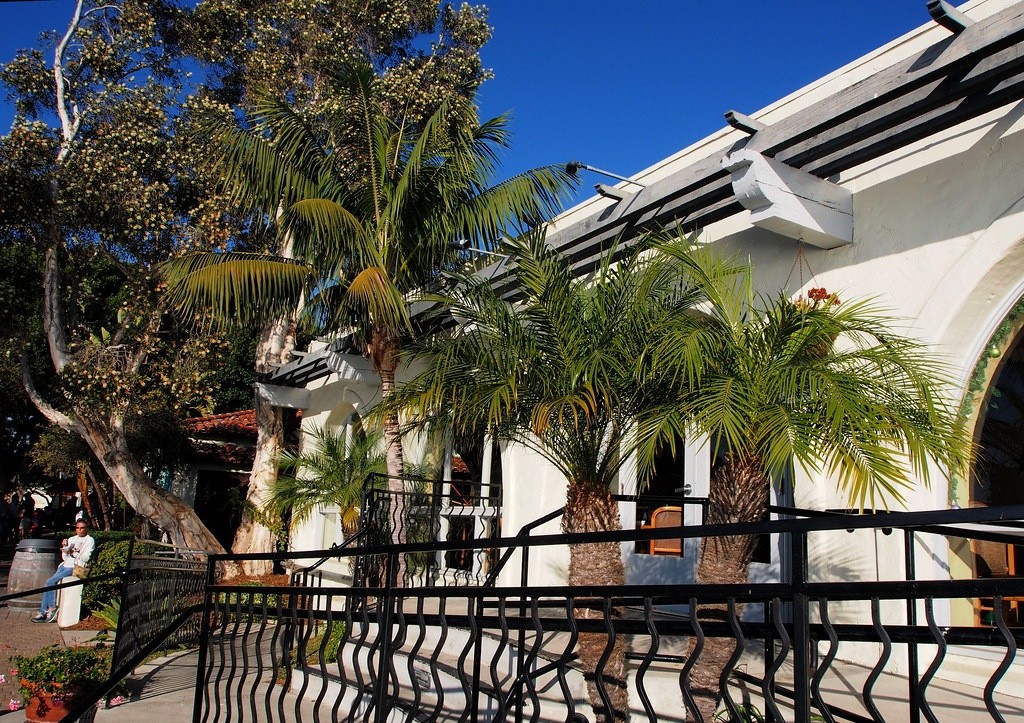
[32, 611, 47, 622]
[45, 607, 60, 624]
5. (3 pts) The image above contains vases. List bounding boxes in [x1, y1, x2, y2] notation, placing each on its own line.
[21, 679, 76, 723]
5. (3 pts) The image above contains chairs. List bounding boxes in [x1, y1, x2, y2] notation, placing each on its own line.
[650, 507, 683, 555]
[971, 501, 1018, 624]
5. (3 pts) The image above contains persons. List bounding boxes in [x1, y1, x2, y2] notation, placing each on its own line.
[31, 518, 94, 622]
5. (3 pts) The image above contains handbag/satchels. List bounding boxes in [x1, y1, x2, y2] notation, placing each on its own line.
[72, 557, 89, 579]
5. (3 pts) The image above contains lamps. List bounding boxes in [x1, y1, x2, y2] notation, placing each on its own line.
[448, 240, 510, 258]
[565, 162, 646, 188]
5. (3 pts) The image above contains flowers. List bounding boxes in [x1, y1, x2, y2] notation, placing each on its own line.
[0, 633, 130, 711]
